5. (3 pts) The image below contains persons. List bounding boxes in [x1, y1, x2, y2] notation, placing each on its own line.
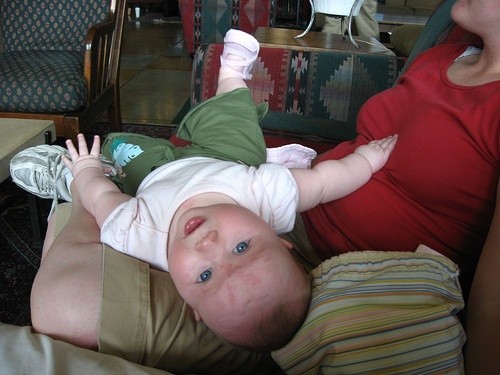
[62, 29, 399, 351]
[9, 0, 500, 375]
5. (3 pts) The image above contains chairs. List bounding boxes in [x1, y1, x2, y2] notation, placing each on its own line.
[0, 0, 128, 151]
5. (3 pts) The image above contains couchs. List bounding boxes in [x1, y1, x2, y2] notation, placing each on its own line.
[168, 0, 497, 275]
[178, 0, 272, 58]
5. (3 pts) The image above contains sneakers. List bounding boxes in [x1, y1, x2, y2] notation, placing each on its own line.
[9, 143, 124, 222]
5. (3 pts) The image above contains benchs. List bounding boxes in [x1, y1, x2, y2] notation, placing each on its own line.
[377, 0, 445, 42]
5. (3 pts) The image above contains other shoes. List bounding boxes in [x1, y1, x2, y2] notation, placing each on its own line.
[219, 28, 260, 81]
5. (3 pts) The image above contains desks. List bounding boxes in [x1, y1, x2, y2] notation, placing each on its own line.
[0, 116, 57, 248]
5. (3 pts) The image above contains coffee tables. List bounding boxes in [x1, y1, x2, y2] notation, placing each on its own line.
[254, 26, 394, 65]
